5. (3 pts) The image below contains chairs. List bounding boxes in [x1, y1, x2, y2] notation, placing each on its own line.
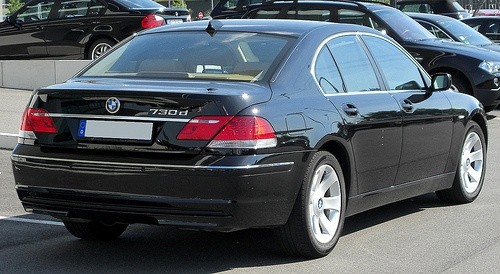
[139, 58, 186, 78]
[179, 48, 232, 71]
[233, 62, 269, 77]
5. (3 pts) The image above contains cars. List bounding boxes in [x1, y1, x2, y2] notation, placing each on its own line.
[406, 11, 499, 64]
[0, 0, 191, 60]
[9, 18, 488, 254]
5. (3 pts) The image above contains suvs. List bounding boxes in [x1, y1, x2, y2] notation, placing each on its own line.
[391, 0, 500, 50]
[208, 0, 500, 112]
